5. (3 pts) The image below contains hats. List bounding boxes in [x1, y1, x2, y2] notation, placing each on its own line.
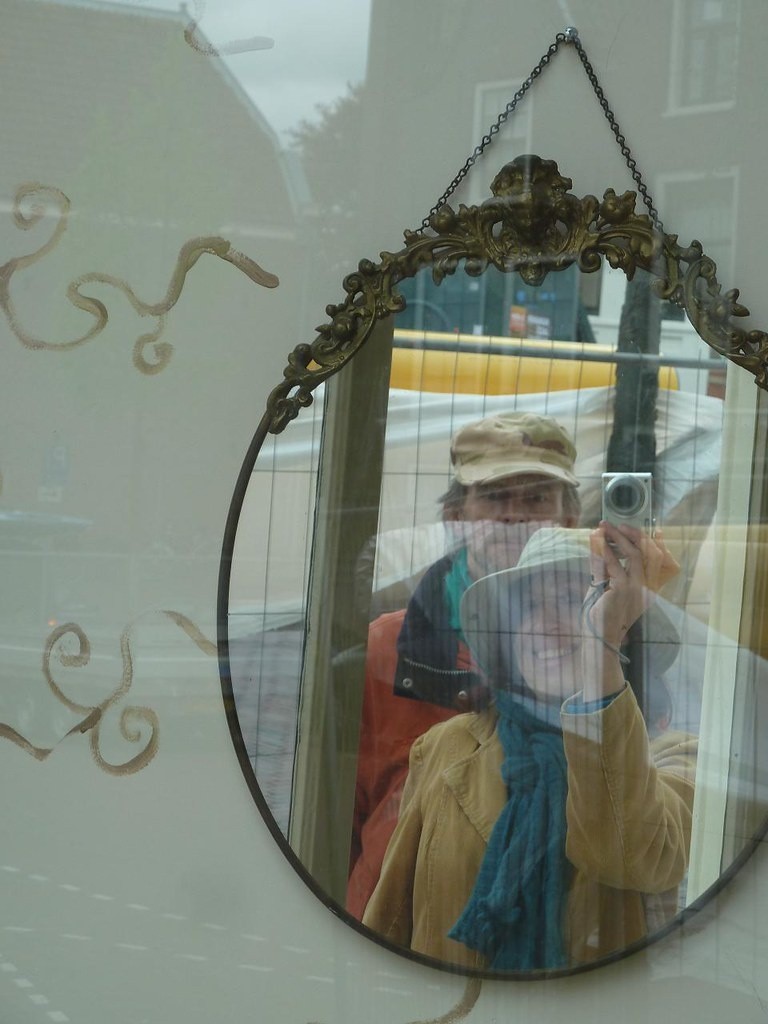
[450, 409, 579, 492]
[458, 525, 680, 687]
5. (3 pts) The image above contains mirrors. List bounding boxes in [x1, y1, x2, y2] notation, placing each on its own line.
[219, 156, 767, 983]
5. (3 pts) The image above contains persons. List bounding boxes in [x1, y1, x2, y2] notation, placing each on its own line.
[346, 411, 699, 969]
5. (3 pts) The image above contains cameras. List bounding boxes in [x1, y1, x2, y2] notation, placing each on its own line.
[602, 471, 655, 550]
